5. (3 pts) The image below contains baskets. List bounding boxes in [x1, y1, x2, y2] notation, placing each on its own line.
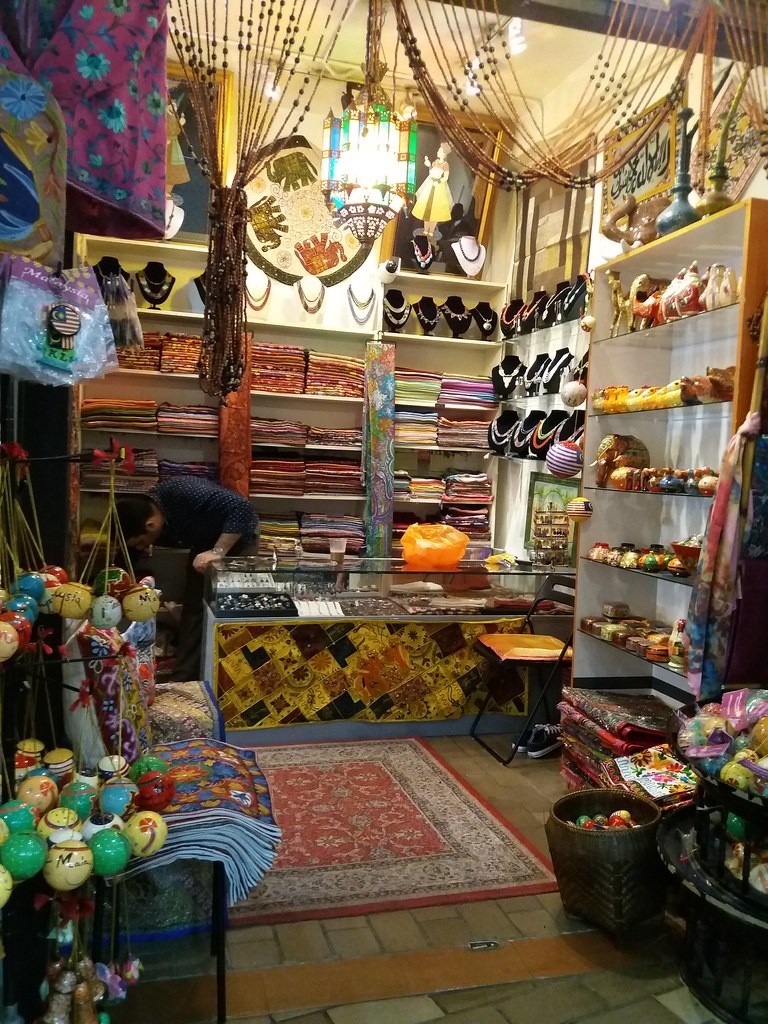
[544, 788, 661, 929]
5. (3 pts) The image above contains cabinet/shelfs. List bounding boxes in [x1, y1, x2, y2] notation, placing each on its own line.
[66, 230, 574, 748]
[111, 859, 231, 1024]
[656, 696, 768, 1024]
[571, 197, 768, 710]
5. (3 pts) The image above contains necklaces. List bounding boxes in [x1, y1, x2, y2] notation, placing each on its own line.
[242, 274, 271, 312]
[383, 282, 606, 333]
[346, 281, 377, 325]
[485, 353, 598, 390]
[410, 238, 483, 269]
[94, 263, 207, 299]
[490, 417, 585, 450]
[167, 203, 175, 229]
[297, 277, 327, 315]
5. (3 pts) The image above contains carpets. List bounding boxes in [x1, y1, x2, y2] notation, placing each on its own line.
[229, 733, 559, 930]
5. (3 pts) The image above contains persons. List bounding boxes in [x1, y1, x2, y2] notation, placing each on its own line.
[110, 472, 261, 681]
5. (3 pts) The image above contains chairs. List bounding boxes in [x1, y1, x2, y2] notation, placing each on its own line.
[469, 573, 576, 766]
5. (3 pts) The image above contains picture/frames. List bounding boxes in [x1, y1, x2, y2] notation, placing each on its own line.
[597, 86, 689, 233]
[166, 59, 236, 245]
[524, 472, 582, 568]
[378, 112, 518, 281]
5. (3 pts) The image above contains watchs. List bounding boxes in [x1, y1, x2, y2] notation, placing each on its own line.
[211, 545, 225, 554]
